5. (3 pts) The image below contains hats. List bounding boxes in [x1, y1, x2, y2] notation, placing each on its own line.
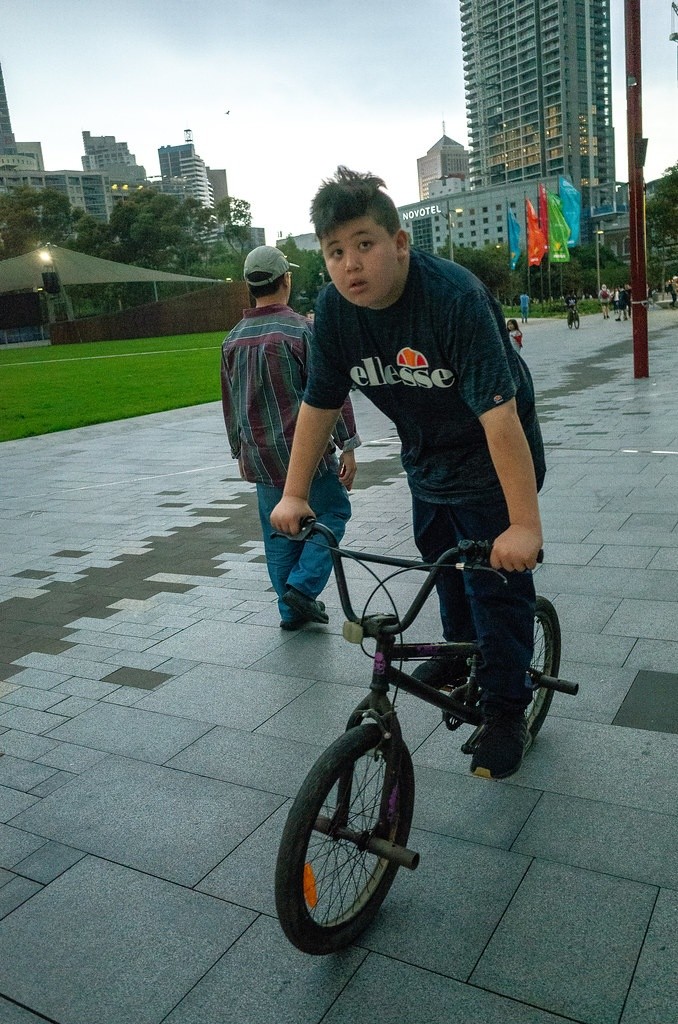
[243, 245, 302, 286]
[601, 284, 607, 291]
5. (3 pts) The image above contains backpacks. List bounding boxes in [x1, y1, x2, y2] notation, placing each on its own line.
[619, 292, 628, 310]
[601, 291, 609, 299]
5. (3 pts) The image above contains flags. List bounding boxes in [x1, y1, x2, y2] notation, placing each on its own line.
[507, 177, 581, 271]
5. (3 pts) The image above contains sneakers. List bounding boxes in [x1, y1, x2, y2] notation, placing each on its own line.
[279, 600, 326, 630]
[282, 589, 330, 624]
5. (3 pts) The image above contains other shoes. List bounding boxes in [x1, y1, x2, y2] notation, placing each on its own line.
[615, 318, 621, 322]
[404, 655, 473, 695]
[623, 317, 627, 321]
[469, 703, 533, 779]
[670, 303, 676, 310]
[603, 314, 610, 319]
[522, 319, 528, 324]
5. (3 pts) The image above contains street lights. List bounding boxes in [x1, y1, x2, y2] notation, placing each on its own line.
[447, 209, 463, 262]
[595, 230, 604, 293]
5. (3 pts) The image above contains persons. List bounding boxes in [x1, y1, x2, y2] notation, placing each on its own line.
[598, 283, 632, 321]
[519, 287, 529, 323]
[506, 319, 523, 354]
[669, 276, 678, 310]
[646, 282, 652, 311]
[270, 166, 548, 781]
[565, 290, 577, 324]
[220, 246, 362, 630]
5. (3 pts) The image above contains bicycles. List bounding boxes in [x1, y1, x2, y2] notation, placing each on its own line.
[267, 515, 581, 955]
[567, 308, 580, 329]
